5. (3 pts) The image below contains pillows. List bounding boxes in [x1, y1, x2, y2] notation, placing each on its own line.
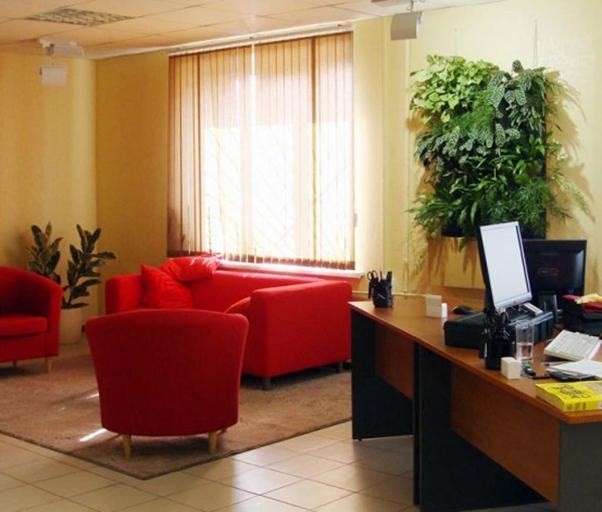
[140, 265, 194, 311]
[161, 256, 220, 280]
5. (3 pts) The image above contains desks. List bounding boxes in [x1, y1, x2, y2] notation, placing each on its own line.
[347, 301, 602, 512]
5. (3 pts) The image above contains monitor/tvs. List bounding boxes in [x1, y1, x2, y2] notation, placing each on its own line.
[522, 240, 586, 324]
[479, 221, 533, 323]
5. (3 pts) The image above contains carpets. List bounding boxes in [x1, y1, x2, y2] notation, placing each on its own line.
[0, 353, 352, 480]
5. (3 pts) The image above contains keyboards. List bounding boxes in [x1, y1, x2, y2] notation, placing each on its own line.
[543, 330, 602, 362]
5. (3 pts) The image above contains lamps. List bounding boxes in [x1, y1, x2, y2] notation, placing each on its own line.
[391, 12, 424, 41]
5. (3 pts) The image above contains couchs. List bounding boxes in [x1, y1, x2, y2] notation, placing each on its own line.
[102, 269, 354, 387]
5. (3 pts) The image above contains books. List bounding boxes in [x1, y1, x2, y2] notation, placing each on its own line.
[534, 380, 601, 412]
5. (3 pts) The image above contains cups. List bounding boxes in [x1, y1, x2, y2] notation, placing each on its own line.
[373, 286, 393, 307]
[515, 319, 535, 362]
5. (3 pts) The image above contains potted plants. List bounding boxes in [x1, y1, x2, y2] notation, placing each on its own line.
[24, 219, 119, 346]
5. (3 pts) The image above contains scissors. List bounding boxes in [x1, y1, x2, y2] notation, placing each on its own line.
[367, 270, 378, 281]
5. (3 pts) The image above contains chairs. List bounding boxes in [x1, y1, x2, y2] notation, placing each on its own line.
[83, 308, 249, 459]
[1, 266, 63, 373]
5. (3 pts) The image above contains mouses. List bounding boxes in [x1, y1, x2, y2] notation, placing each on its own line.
[453, 305, 474, 315]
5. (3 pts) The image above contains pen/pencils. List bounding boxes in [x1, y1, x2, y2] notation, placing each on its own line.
[380, 271, 391, 284]
[543, 362, 563, 365]
